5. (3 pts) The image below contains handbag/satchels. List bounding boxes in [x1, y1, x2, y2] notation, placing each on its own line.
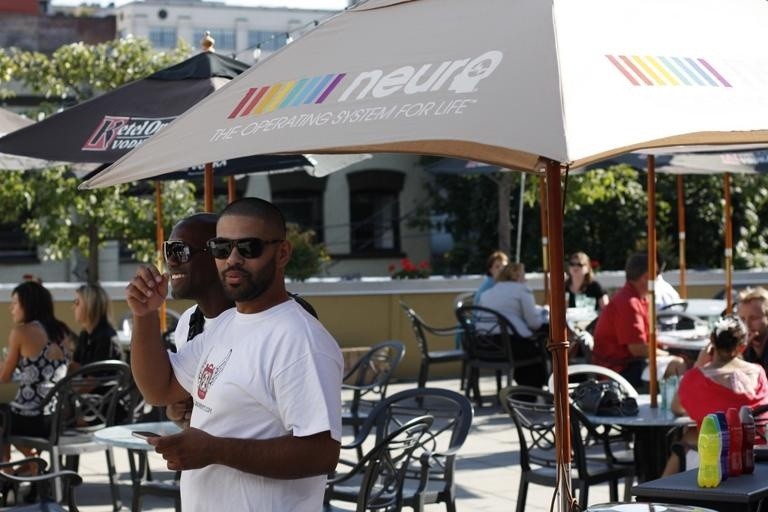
[573, 376, 640, 418]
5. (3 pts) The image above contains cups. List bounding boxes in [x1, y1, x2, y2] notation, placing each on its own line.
[693, 316, 719, 341]
[565, 291, 596, 316]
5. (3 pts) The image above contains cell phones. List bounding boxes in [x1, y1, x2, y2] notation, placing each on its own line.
[131, 431, 161, 441]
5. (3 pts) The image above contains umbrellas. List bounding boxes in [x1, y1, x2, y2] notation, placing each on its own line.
[79, 151, 374, 214]
[0, 31, 370, 214]
[82, 1, 768, 511]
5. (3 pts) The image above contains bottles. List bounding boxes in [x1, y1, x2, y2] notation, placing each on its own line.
[660, 375, 684, 412]
[698, 407, 756, 487]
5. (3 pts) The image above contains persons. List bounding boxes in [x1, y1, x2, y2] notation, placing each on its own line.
[124, 195, 344, 511]
[470, 251, 511, 331]
[54, 280, 121, 483]
[0, 280, 72, 477]
[163, 211, 322, 431]
[734, 286, 767, 374]
[643, 246, 681, 316]
[560, 248, 609, 363]
[473, 263, 545, 396]
[660, 315, 768, 478]
[593, 253, 690, 394]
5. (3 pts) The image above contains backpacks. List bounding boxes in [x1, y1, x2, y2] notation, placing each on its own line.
[187, 291, 320, 344]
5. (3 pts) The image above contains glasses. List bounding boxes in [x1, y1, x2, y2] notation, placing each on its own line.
[569, 261, 589, 268]
[205, 235, 284, 260]
[159, 239, 206, 266]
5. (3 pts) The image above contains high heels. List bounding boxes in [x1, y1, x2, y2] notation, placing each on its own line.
[0, 472, 50, 506]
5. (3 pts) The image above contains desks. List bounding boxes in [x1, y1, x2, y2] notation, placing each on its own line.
[92, 420, 189, 512]
[569, 393, 699, 486]
[630, 461, 768, 512]
[654, 328, 711, 362]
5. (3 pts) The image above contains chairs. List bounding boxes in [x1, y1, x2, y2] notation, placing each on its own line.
[499, 384, 646, 512]
[321, 339, 472, 512]
[548, 363, 639, 504]
[657, 301, 702, 330]
[400, 293, 550, 414]
[0, 359, 152, 512]
